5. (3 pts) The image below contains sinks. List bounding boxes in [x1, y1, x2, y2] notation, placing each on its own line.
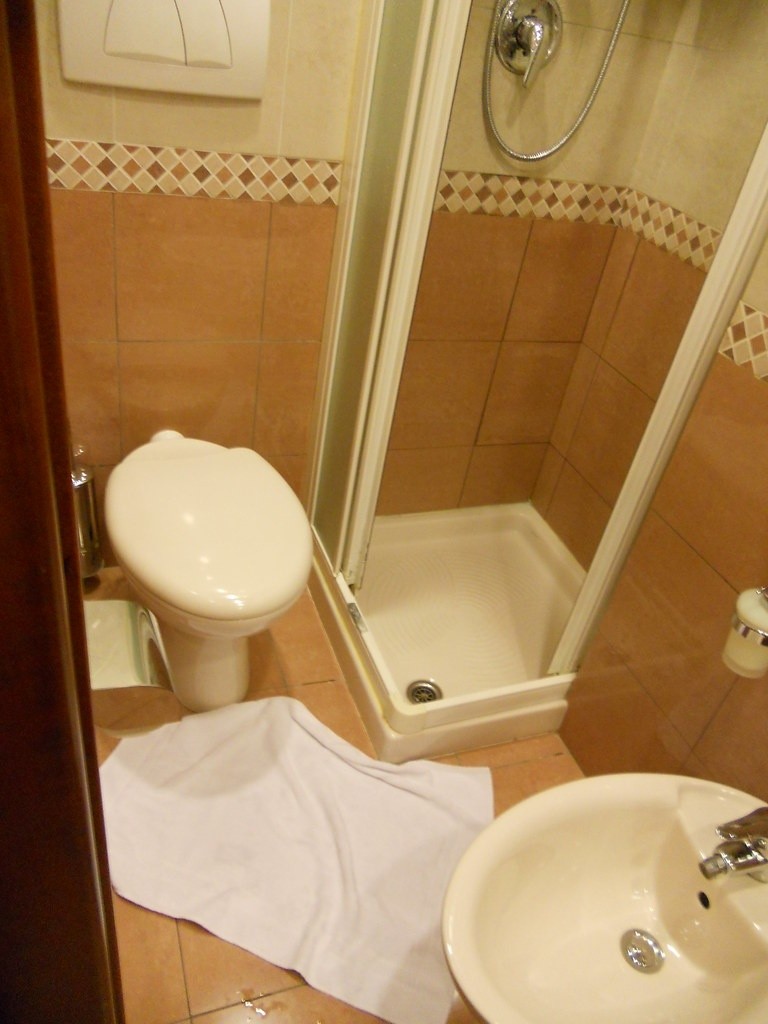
[441, 772, 767, 1024]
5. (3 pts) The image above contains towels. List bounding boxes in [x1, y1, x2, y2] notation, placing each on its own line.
[100, 694, 495, 1023]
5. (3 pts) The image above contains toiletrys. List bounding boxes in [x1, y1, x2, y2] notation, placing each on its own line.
[721, 585, 768, 679]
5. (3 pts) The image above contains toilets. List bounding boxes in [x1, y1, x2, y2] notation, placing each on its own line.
[103, 428, 314, 712]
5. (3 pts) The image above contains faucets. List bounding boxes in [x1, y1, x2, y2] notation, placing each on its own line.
[697, 806, 768, 883]
